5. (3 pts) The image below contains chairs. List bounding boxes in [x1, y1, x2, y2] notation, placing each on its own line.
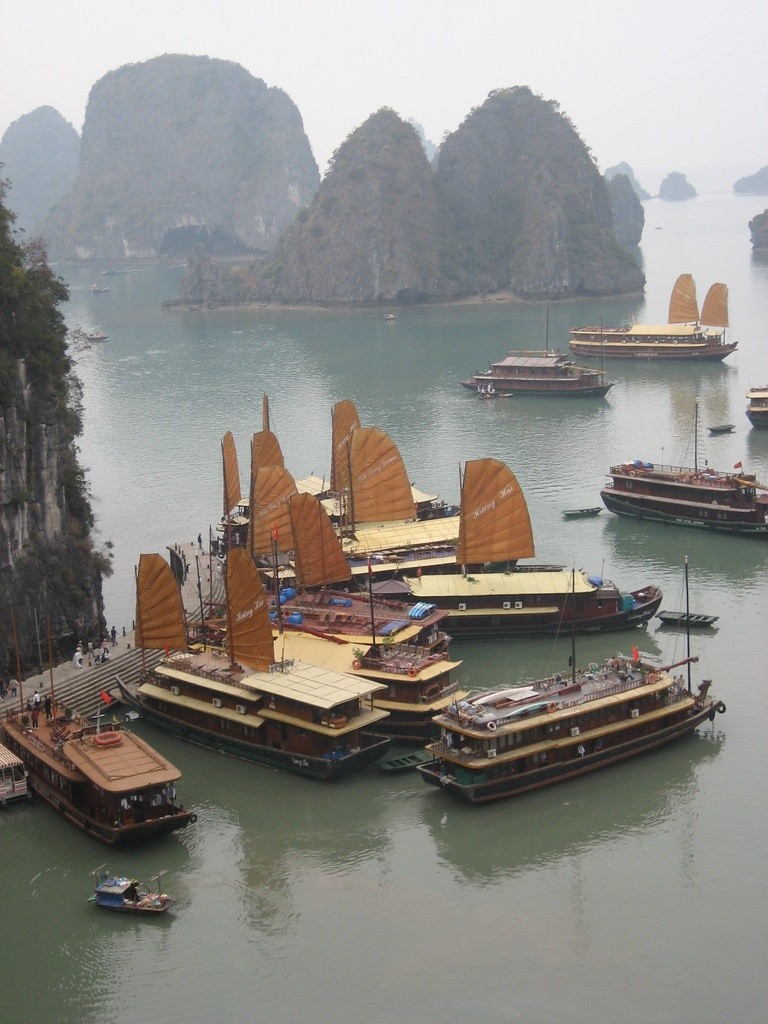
[317, 610, 387, 630]
[50, 726, 72, 744]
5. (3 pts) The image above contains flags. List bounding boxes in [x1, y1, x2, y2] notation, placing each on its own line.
[270, 523, 279, 541]
[632, 645, 639, 664]
[101, 687, 111, 705]
[368, 559, 373, 579]
[417, 566, 423, 580]
[164, 642, 169, 657]
[734, 462, 741, 468]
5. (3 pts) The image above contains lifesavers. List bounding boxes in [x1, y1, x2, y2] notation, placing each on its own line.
[648, 674, 655, 683]
[407, 666, 416, 677]
[352, 659, 362, 670]
[487, 722, 497, 731]
[95, 731, 122, 745]
[718, 703, 726, 713]
[547, 702, 556, 713]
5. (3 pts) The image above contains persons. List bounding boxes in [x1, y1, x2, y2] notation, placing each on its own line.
[31, 708, 39, 730]
[94, 650, 110, 664]
[197, 534, 203, 551]
[88, 640, 96, 660]
[73, 650, 84, 669]
[0, 677, 8, 702]
[576, 657, 686, 701]
[33, 691, 42, 712]
[130, 879, 145, 902]
[175, 804, 188, 815]
[26, 699, 33, 718]
[102, 639, 109, 654]
[9, 677, 19, 699]
[76, 640, 85, 654]
[45, 695, 53, 722]
[111, 626, 119, 647]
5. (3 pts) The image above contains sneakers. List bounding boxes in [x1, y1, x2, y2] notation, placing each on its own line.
[32, 727, 35, 729]
[35, 727, 38, 729]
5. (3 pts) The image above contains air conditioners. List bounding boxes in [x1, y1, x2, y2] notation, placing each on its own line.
[433, 633, 437, 641]
[364, 693, 371, 700]
[570, 727, 579, 737]
[332, 523, 338, 528]
[430, 500, 441, 509]
[515, 602, 522, 608]
[236, 705, 245, 714]
[171, 687, 179, 695]
[413, 635, 418, 641]
[741, 489, 745, 494]
[630, 709, 639, 719]
[427, 636, 432, 644]
[212, 699, 222, 707]
[504, 602, 510, 609]
[459, 604, 466, 611]
[486, 749, 496, 758]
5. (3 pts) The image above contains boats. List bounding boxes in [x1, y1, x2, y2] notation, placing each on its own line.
[0, 743, 29, 806]
[568, 282, 738, 362]
[416, 555, 727, 802]
[567, 273, 711, 345]
[562, 507, 604, 519]
[108, 553, 393, 781]
[376, 747, 442, 774]
[477, 390, 514, 399]
[0, 601, 198, 849]
[745, 385, 768, 430]
[183, 392, 518, 746]
[390, 458, 664, 637]
[459, 303, 615, 398]
[599, 395, 768, 536]
[87, 864, 176, 913]
[707, 424, 735, 433]
[655, 609, 720, 626]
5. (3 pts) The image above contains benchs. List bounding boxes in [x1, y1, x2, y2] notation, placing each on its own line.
[683, 614, 704, 620]
